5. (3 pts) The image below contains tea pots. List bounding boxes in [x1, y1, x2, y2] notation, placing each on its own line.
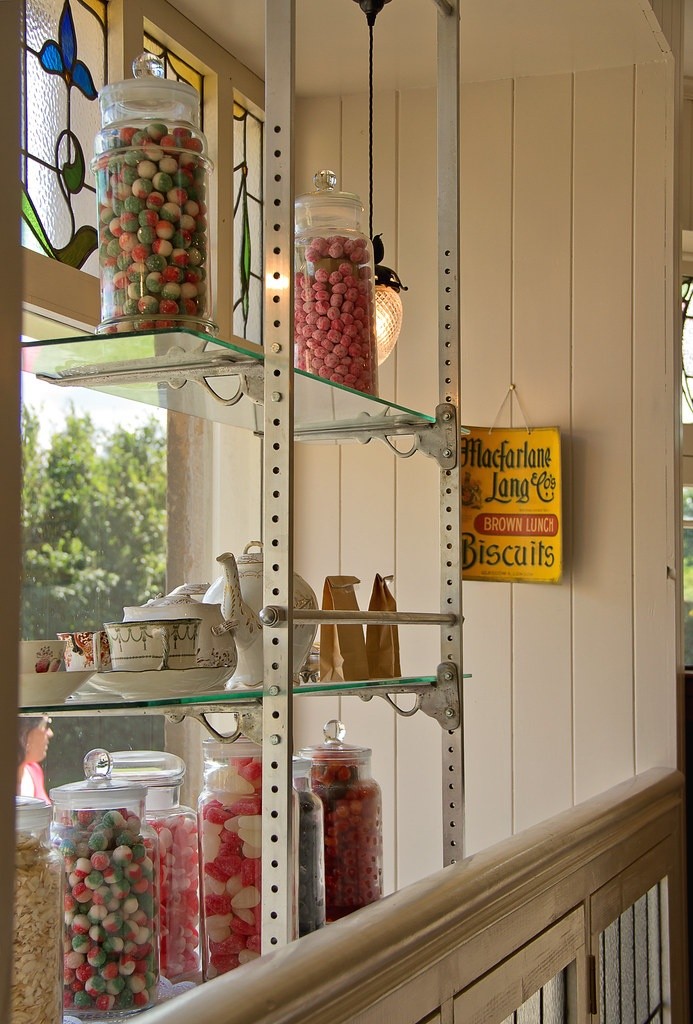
[202, 541, 319, 689]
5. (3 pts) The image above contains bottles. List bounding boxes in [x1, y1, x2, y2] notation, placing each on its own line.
[168, 583, 211, 602]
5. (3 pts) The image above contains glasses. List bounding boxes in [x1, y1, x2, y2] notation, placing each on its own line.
[39, 718, 52, 730]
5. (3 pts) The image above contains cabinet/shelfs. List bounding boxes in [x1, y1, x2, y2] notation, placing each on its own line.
[16, 0, 468, 1024]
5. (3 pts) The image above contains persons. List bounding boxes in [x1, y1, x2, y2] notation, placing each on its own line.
[17, 718, 54, 810]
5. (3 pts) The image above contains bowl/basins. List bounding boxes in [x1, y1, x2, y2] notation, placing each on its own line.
[56, 632, 112, 672]
[103, 618, 203, 671]
[18, 670, 99, 705]
[122, 593, 238, 692]
[19, 640, 67, 672]
[88, 667, 235, 699]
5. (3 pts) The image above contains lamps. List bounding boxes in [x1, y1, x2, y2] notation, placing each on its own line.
[349, 0, 411, 365]
